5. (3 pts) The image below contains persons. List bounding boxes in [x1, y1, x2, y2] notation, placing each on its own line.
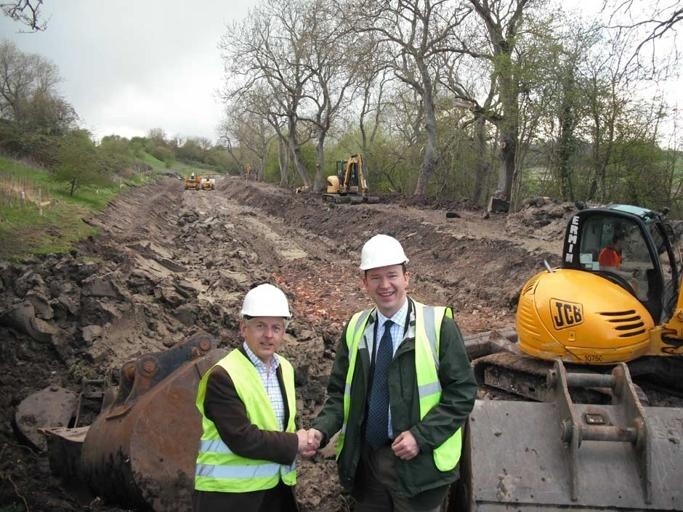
[294, 233, 480, 512]
[207, 177, 210, 181]
[598, 227, 641, 296]
[190, 169, 195, 179]
[191, 282, 319, 512]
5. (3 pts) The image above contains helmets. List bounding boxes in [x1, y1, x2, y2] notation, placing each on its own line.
[240, 283, 292, 320]
[359, 233, 410, 271]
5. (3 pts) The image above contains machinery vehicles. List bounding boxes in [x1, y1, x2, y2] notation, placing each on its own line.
[183, 168, 200, 190]
[456, 203, 681, 407]
[200, 178, 215, 190]
[321, 153, 380, 205]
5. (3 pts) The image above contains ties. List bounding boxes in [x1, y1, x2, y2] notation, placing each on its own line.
[365, 322, 395, 451]
